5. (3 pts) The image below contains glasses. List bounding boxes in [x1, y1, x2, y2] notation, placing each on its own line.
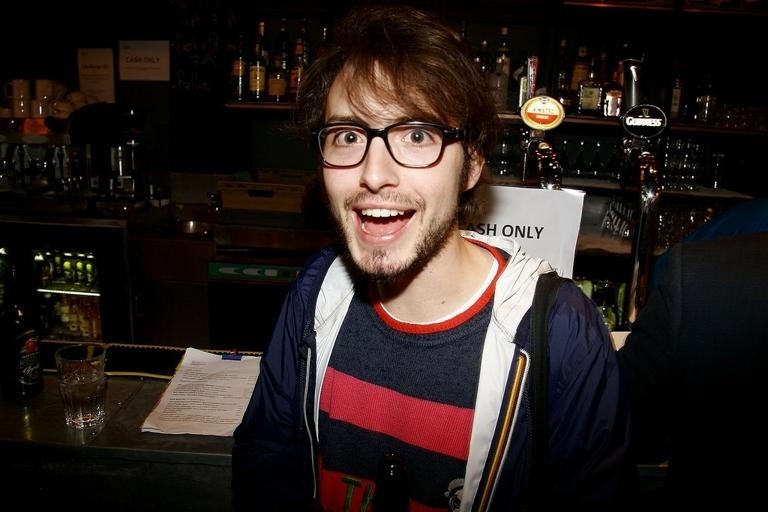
[310, 115, 471, 172]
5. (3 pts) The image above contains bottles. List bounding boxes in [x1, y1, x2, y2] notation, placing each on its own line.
[456, 18, 753, 129]
[575, 274, 619, 331]
[47, 248, 103, 344]
[232, 16, 331, 104]
[0, 244, 46, 408]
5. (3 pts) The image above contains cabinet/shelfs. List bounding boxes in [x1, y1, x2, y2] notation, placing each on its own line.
[189, 85, 749, 288]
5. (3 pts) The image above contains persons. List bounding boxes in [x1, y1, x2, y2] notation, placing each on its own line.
[614, 198, 766, 512]
[230, 5, 620, 511]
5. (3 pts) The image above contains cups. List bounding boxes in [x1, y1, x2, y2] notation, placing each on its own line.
[55, 343, 108, 429]
[491, 128, 724, 192]
[600, 198, 714, 248]
[1, 76, 64, 119]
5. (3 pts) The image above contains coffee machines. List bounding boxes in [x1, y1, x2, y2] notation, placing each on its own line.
[87, 129, 149, 211]
